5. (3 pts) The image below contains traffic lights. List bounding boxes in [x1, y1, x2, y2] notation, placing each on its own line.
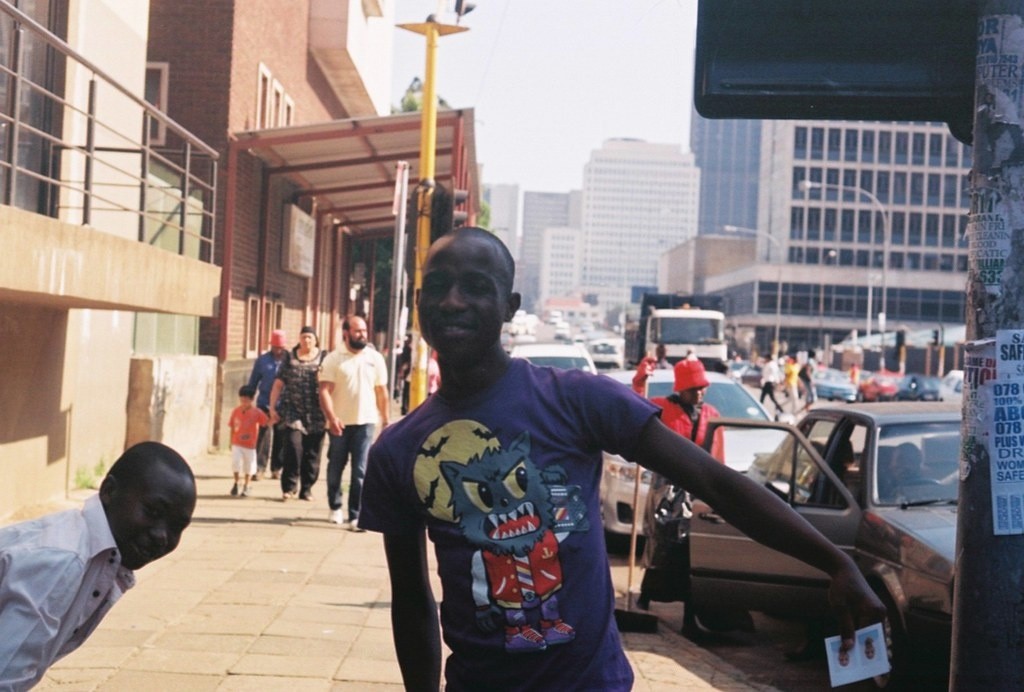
[431, 176, 469, 246]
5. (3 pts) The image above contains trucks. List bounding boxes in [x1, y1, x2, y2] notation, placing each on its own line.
[624, 290, 729, 370]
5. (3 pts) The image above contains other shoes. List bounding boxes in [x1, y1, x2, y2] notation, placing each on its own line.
[241, 486, 248, 496]
[302, 495, 313, 502]
[230, 485, 237, 494]
[635, 595, 650, 608]
[782, 638, 826, 659]
[283, 491, 294, 502]
[348, 520, 366, 532]
[739, 613, 757, 632]
[328, 509, 344, 525]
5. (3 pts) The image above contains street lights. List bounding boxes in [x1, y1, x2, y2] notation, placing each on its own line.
[724, 225, 784, 355]
[798, 180, 884, 372]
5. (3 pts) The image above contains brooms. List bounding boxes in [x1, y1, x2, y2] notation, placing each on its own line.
[610, 362, 660, 637]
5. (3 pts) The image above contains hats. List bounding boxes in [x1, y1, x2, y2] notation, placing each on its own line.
[271, 329, 287, 348]
[673, 360, 710, 392]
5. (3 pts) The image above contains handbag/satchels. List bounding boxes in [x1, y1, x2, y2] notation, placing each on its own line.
[798, 367, 810, 381]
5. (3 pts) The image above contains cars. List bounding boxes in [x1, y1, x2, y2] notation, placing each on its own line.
[728, 357, 964, 404]
[688, 405, 961, 692]
[499, 304, 621, 374]
[593, 373, 774, 555]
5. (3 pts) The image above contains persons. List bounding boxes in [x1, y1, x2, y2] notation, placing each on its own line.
[631, 353, 725, 644]
[226, 385, 278, 499]
[831, 634, 855, 669]
[781, 354, 798, 418]
[798, 348, 818, 416]
[358, 225, 884, 692]
[880, 442, 941, 498]
[758, 352, 785, 414]
[0, 441, 197, 692]
[270, 326, 330, 503]
[651, 344, 674, 372]
[248, 328, 289, 480]
[857, 631, 878, 660]
[318, 314, 389, 532]
